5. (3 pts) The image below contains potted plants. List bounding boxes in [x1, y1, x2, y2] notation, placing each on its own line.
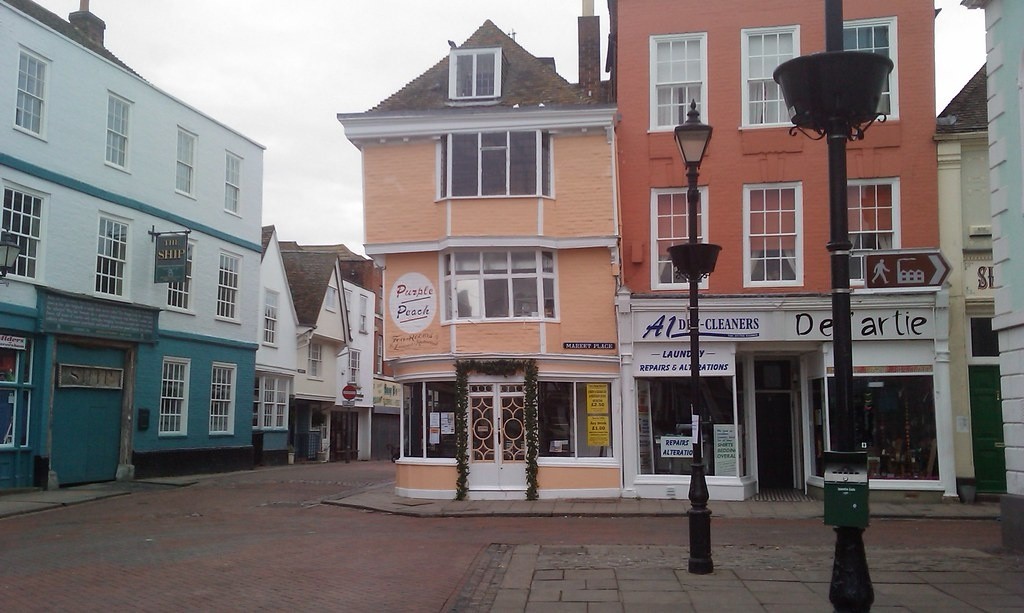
[288, 443, 296, 464]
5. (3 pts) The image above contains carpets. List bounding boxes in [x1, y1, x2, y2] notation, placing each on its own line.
[754, 488, 816, 502]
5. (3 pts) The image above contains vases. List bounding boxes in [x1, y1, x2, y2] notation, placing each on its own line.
[317, 451, 328, 461]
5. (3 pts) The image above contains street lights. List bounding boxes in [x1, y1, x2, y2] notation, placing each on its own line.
[666, 100, 722, 576]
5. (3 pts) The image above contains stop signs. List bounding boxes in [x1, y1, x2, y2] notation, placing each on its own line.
[342, 386, 357, 399]
